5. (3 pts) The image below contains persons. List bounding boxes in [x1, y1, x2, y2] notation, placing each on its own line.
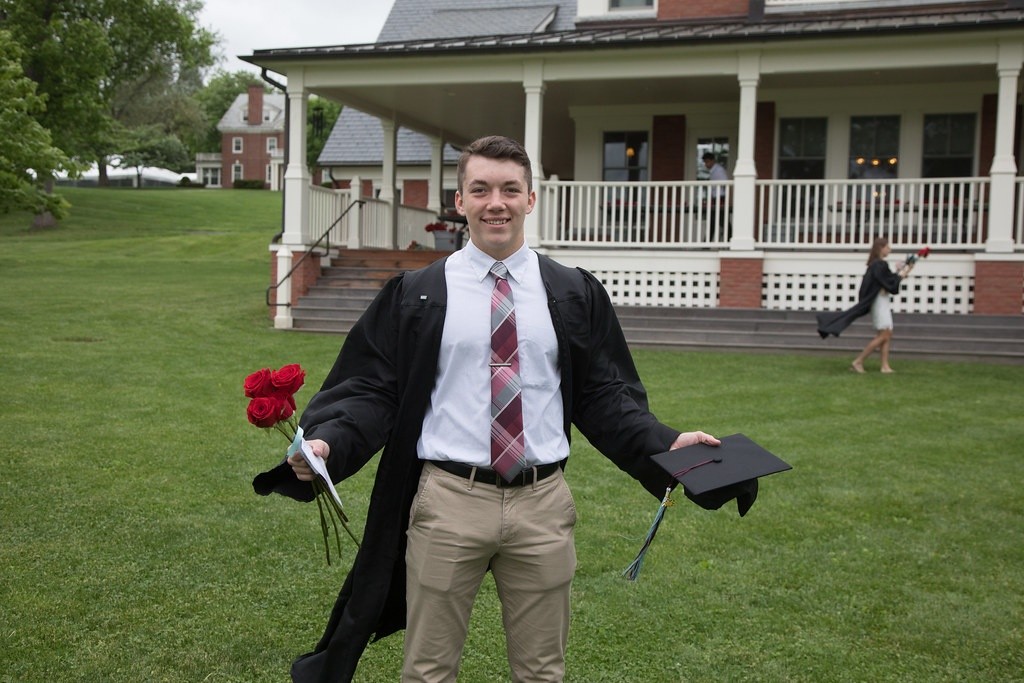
[851, 238, 911, 374]
[701, 152, 732, 251]
[286, 136, 722, 683]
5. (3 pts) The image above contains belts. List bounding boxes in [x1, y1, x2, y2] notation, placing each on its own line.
[428, 460, 559, 489]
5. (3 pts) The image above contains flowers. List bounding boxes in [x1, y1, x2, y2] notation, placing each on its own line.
[244, 363, 362, 572]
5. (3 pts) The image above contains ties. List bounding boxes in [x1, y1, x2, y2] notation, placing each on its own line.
[488, 262, 525, 484]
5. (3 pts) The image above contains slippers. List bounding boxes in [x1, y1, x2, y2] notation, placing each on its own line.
[848, 360, 867, 374]
[880, 367, 896, 373]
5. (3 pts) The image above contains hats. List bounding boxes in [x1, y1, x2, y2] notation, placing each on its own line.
[619, 433, 794, 582]
